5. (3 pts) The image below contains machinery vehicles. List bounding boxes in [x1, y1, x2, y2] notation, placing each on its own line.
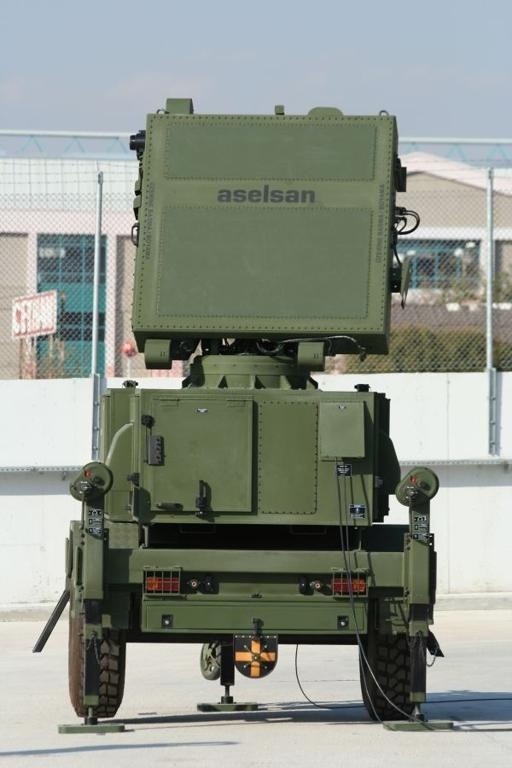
[32, 95, 460, 731]
